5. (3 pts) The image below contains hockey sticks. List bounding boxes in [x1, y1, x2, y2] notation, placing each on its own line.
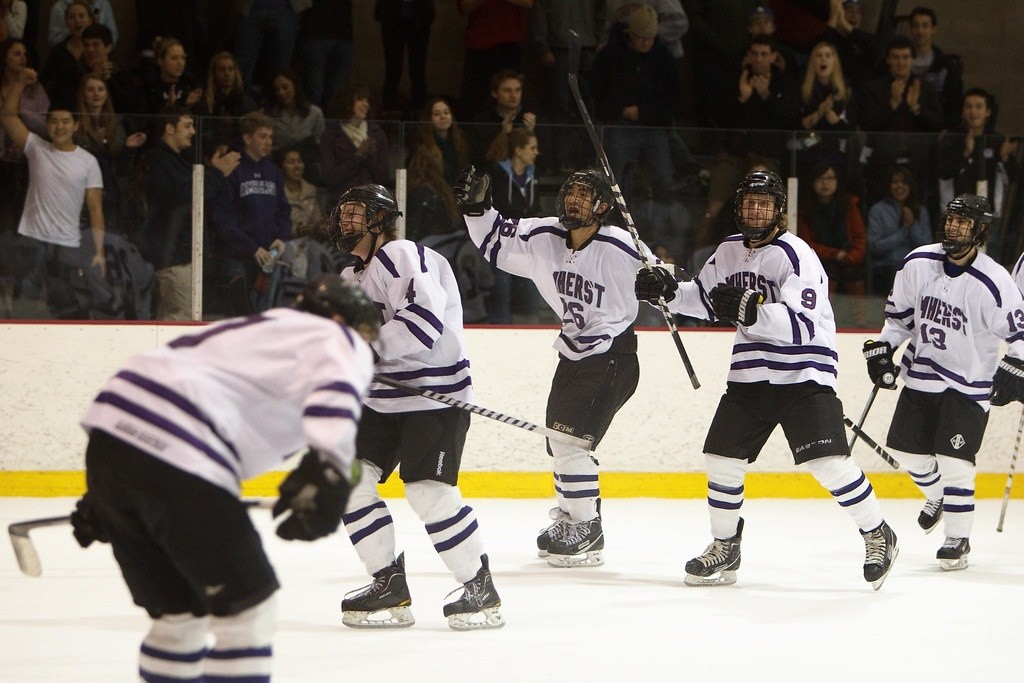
[997, 405, 1024, 532]
[374, 372, 592, 451]
[848, 337, 911, 453]
[8, 499, 317, 576]
[655, 264, 900, 469]
[566, 28, 701, 390]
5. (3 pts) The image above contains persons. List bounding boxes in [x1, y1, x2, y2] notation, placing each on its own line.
[0, 0, 1024, 328]
[634, 168, 900, 590]
[451, 163, 690, 567]
[332, 186, 508, 631]
[69, 275, 384, 683]
[863, 192, 1023, 571]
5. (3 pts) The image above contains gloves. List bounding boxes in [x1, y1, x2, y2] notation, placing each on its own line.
[987, 353, 1024, 406]
[862, 339, 900, 391]
[69, 491, 112, 549]
[272, 448, 352, 540]
[452, 163, 492, 212]
[634, 265, 678, 306]
[708, 283, 759, 326]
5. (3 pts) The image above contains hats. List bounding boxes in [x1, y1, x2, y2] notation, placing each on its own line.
[745, 4, 773, 20]
[628, 3, 659, 37]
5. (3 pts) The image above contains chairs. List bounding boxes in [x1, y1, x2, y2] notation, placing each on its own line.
[841, 158, 931, 297]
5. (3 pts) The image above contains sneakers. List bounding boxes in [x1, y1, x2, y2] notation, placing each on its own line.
[859, 520, 899, 591]
[918, 495, 944, 534]
[442, 553, 505, 630]
[936, 537, 971, 571]
[536, 498, 606, 565]
[339, 550, 415, 628]
[683, 516, 744, 585]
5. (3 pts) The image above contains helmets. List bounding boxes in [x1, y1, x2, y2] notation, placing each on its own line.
[555, 165, 615, 230]
[325, 183, 403, 253]
[732, 169, 786, 243]
[294, 270, 372, 323]
[938, 193, 992, 255]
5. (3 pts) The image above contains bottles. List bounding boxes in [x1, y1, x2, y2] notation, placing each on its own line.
[261, 248, 278, 271]
